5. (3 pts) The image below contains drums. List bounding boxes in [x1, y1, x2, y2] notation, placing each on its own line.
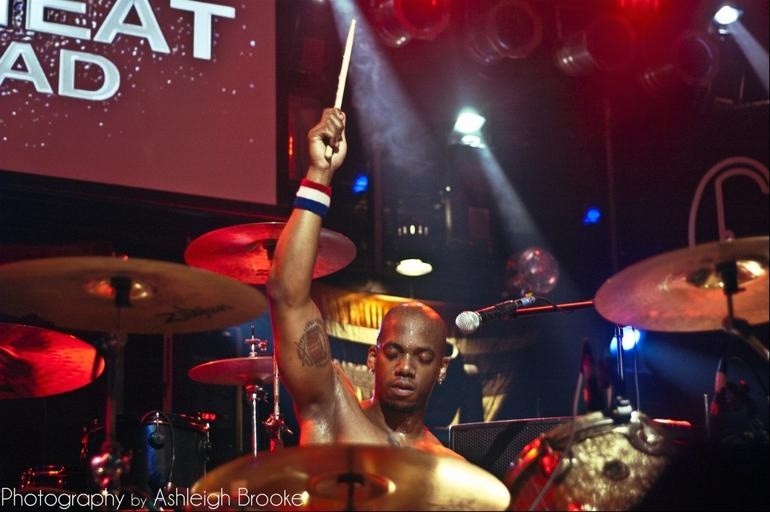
[81, 413, 209, 492]
[506, 405, 688, 512]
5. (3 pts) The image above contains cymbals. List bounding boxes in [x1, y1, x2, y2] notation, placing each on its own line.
[183, 221, 357, 288]
[188, 354, 279, 387]
[4, 255, 272, 336]
[189, 444, 512, 511]
[1, 322, 109, 400]
[592, 236, 768, 333]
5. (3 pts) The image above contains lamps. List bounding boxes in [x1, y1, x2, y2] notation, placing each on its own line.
[364, 0, 721, 99]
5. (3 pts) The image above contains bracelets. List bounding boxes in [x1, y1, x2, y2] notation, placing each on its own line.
[292, 172, 333, 217]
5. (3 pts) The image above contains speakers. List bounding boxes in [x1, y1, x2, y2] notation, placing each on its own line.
[450, 417, 582, 483]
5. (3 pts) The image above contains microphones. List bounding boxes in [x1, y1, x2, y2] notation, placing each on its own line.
[455, 293, 536, 334]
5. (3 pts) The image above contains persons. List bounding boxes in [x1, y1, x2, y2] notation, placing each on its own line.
[267, 107, 464, 459]
